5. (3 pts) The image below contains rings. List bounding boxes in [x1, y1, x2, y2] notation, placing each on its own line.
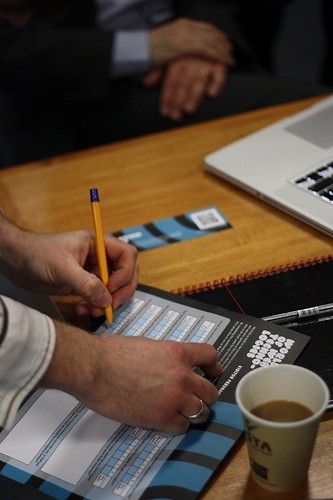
[198, 66, 210, 76]
[183, 399, 206, 420]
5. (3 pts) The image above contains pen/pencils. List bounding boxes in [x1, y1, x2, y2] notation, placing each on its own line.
[90, 187, 112, 328]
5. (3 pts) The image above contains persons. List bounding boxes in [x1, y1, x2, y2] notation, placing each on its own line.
[0, 213, 223, 435]
[0, 0, 241, 136]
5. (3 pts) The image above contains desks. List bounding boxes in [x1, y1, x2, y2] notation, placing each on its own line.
[0, 95, 333, 500]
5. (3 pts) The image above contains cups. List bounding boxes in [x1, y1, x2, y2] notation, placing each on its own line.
[234, 363, 330, 494]
